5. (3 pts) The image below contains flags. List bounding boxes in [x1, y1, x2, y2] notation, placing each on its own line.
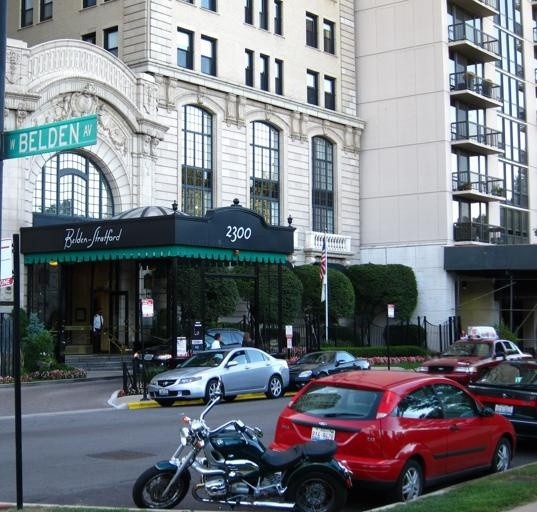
[319, 237, 327, 303]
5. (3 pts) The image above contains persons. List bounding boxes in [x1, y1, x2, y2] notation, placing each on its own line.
[468, 328, 480, 339]
[92, 306, 105, 354]
[210, 333, 224, 364]
[460, 329, 467, 341]
[240, 332, 253, 355]
